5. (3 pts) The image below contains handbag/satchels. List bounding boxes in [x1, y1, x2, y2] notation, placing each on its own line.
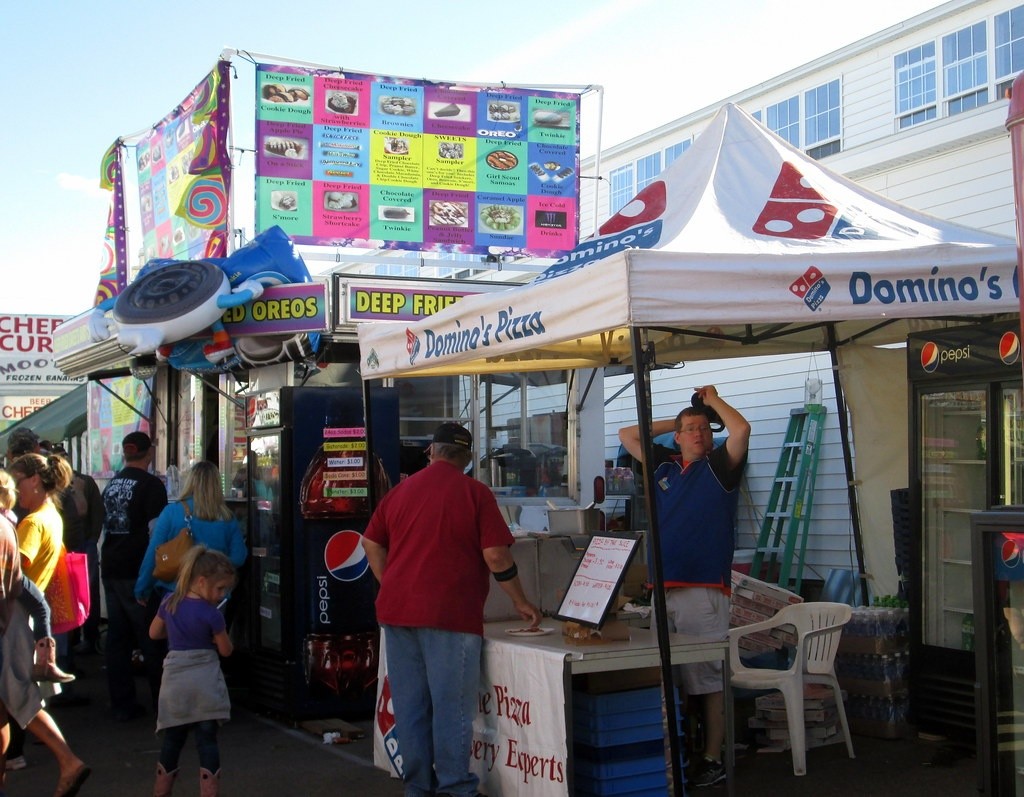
[154, 499, 194, 584]
[45, 545, 92, 635]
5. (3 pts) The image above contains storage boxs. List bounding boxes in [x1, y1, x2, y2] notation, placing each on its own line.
[732, 549, 780, 582]
[729, 570, 804, 659]
[574, 749, 690, 797]
[573, 685, 685, 749]
[562, 563, 652, 640]
[842, 718, 915, 740]
[836, 675, 910, 702]
[838, 634, 909, 655]
[548, 509, 602, 538]
[747, 688, 852, 753]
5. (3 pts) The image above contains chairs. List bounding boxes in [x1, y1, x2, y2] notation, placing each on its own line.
[729, 602, 857, 776]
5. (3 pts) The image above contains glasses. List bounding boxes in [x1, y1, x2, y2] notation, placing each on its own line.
[677, 424, 712, 434]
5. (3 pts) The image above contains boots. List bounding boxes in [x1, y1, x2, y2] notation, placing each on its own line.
[153, 762, 181, 797]
[33, 636, 77, 683]
[200, 767, 220, 797]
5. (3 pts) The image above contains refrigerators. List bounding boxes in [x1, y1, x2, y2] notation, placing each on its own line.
[969, 502, 1023, 796]
[903, 323, 1023, 743]
[243, 386, 403, 724]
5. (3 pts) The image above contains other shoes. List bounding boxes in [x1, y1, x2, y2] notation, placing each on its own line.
[112, 701, 147, 718]
[78, 638, 101, 654]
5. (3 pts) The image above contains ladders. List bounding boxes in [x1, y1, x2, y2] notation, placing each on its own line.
[744, 407, 827, 593]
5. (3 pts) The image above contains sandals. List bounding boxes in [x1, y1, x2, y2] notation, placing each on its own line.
[55, 764, 93, 797]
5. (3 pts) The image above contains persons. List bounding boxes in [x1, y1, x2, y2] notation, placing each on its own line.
[0, 467, 77, 684]
[53, 446, 106, 673]
[617, 385, 752, 790]
[147, 544, 237, 797]
[102, 431, 169, 678]
[0, 451, 94, 796]
[361, 421, 543, 797]
[4, 427, 45, 526]
[130, 460, 250, 735]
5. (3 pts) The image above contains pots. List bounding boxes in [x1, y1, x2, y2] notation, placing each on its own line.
[545, 508, 601, 534]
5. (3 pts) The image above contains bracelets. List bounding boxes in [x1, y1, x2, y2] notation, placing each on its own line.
[492, 562, 520, 583]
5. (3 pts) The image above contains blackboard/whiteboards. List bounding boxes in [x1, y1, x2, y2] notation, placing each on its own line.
[551, 530, 644, 630]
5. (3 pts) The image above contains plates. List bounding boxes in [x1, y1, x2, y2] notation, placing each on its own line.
[504, 626, 555, 636]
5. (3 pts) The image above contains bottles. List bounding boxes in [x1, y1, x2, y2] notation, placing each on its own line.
[837, 590, 906, 723]
[607, 466, 634, 496]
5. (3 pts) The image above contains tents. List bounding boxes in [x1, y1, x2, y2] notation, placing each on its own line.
[353, 101, 1023, 797]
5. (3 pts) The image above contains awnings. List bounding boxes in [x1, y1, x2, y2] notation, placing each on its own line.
[1, 383, 91, 461]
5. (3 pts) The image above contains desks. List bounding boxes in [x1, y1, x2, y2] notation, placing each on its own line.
[373, 611, 736, 797]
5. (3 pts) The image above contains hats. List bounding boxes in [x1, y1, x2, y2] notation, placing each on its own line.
[122, 432, 151, 461]
[691, 393, 725, 432]
[424, 422, 473, 453]
[8, 427, 39, 454]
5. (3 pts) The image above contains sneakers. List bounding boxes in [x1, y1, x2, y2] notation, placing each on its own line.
[5, 755, 27, 770]
[689, 757, 729, 787]
